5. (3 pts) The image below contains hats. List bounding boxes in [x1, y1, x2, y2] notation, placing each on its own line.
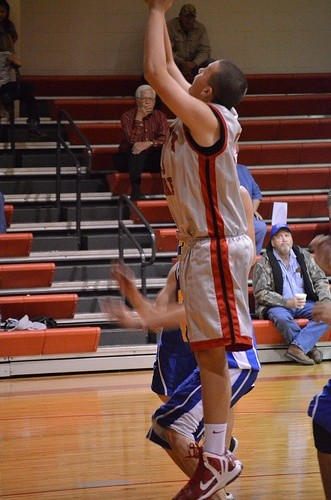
[180, 3, 197, 16]
[270, 224, 290, 236]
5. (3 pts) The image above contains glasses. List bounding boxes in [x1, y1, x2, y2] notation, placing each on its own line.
[137, 97, 155, 100]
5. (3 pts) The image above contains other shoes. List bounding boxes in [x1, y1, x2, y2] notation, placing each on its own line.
[285, 344, 315, 364]
[130, 191, 145, 201]
[230, 436, 238, 452]
[308, 345, 323, 364]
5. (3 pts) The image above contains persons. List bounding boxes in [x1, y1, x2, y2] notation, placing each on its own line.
[0, 51, 47, 137]
[114, 85, 170, 200]
[236, 165, 267, 256]
[142, 0, 254, 500]
[309, 222, 331, 276]
[167, 4, 216, 75]
[100, 186, 261, 500]
[253, 224, 331, 365]
[308, 302, 331, 500]
[0, 0, 18, 50]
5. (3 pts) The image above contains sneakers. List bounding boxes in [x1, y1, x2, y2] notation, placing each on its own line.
[171, 451, 242, 500]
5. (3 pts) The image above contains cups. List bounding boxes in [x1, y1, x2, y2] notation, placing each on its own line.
[132, 141, 141, 155]
[295, 293, 307, 310]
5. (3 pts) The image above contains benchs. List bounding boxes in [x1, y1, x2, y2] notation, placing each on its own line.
[0, 73, 331, 365]
